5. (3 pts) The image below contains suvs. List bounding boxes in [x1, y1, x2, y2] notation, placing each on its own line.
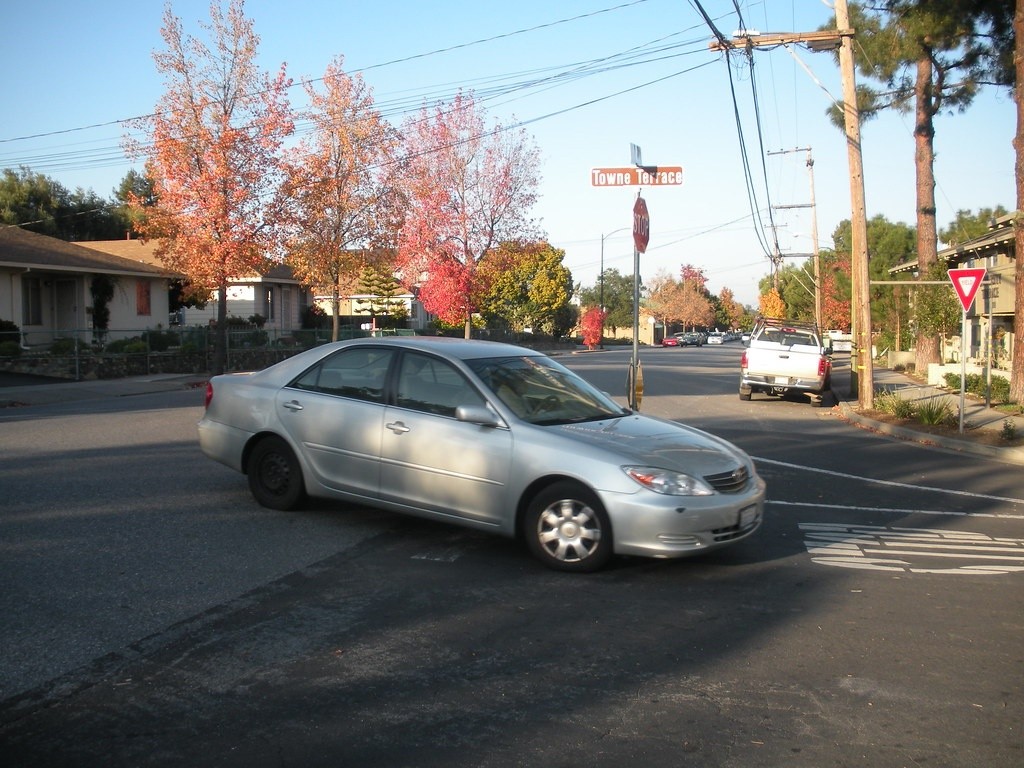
[680, 332, 703, 347]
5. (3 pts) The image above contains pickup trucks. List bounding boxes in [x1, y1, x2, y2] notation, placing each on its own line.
[739, 318, 833, 407]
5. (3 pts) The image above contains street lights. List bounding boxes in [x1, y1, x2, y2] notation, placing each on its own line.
[683, 269, 708, 333]
[600, 227, 633, 349]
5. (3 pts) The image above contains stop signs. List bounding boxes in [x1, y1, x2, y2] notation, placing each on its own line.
[633, 197, 650, 253]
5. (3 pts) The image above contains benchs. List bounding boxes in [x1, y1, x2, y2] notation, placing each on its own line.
[316, 368, 387, 400]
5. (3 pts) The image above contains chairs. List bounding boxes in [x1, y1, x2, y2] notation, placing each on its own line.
[398, 375, 423, 407]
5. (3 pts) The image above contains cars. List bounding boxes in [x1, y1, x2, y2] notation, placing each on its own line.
[707, 333, 723, 345]
[196, 335, 765, 574]
[822, 330, 842, 339]
[703, 332, 708, 342]
[724, 332, 751, 342]
[742, 333, 751, 345]
[699, 332, 706, 344]
[662, 335, 680, 347]
[674, 332, 685, 342]
